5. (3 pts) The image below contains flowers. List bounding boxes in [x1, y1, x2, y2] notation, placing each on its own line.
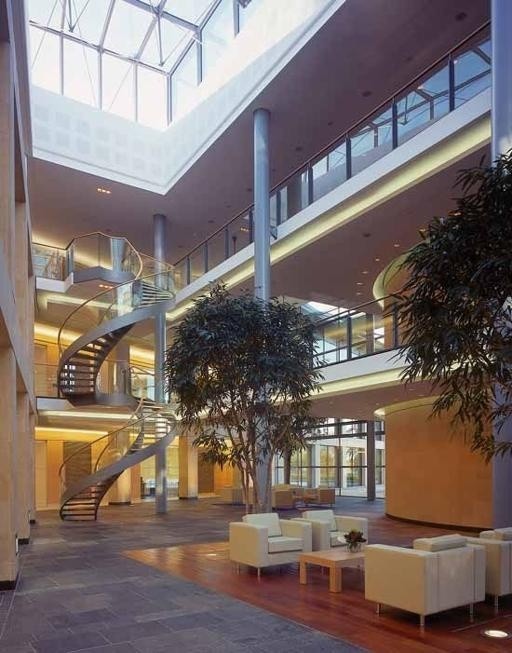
[343, 528, 367, 550]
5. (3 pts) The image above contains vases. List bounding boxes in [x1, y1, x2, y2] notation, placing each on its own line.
[347, 543, 361, 552]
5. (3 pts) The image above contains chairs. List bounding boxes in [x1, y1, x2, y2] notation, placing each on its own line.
[463, 527, 512, 613]
[363, 533, 486, 625]
[290, 509, 368, 575]
[227, 513, 312, 579]
